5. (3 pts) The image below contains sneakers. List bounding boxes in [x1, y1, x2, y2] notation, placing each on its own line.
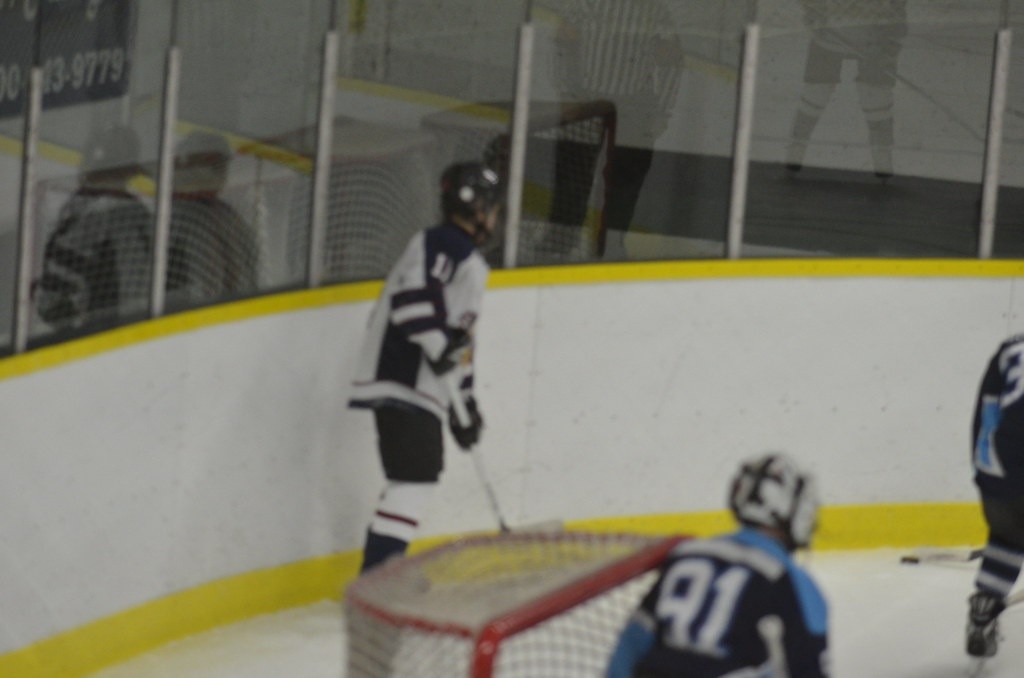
[966, 594, 1004, 678]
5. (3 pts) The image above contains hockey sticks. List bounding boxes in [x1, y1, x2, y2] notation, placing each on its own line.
[900, 549, 986, 566]
[442, 371, 566, 536]
[823, 28, 987, 145]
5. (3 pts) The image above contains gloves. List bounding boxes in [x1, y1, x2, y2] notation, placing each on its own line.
[449, 398, 482, 449]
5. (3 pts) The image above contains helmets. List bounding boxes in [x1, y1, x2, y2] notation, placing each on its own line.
[179, 132, 234, 195]
[84, 125, 143, 181]
[439, 161, 499, 216]
[730, 453, 820, 544]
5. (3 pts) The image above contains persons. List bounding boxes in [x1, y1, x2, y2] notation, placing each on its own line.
[543, 0, 684, 258]
[962, 333, 1023, 660]
[782, 0, 910, 203]
[31, 128, 256, 330]
[344, 160, 502, 677]
[600, 445, 830, 678]
